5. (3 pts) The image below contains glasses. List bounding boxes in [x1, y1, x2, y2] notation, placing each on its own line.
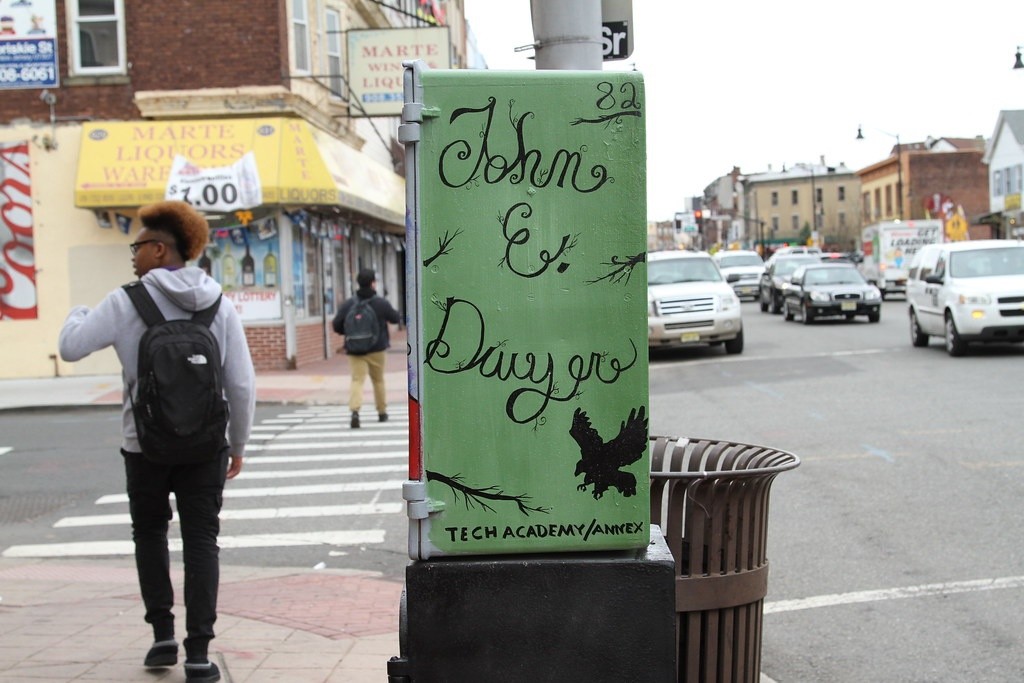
[130, 239, 167, 256]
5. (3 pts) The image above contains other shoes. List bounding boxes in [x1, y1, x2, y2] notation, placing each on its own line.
[379, 414, 387, 422]
[184, 662, 220, 683]
[351, 414, 359, 428]
[144, 641, 178, 666]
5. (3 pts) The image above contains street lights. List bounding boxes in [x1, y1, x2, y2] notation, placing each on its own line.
[855, 124, 904, 221]
[781, 162, 818, 231]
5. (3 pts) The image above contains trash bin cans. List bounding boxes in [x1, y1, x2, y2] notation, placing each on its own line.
[651, 435, 800, 683]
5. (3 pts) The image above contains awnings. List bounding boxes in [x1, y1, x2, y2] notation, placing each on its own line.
[75, 117, 406, 236]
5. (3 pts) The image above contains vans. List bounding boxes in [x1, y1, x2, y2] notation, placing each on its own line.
[905, 238, 1024, 358]
[856, 220, 945, 302]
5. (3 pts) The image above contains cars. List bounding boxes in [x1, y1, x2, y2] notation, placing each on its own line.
[757, 245, 884, 325]
[711, 249, 764, 305]
[647, 250, 744, 361]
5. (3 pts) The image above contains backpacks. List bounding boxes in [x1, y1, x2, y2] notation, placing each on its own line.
[345, 292, 379, 355]
[121, 280, 229, 463]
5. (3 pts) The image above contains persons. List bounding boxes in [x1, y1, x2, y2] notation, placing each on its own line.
[60, 202, 257, 683]
[333, 269, 400, 428]
